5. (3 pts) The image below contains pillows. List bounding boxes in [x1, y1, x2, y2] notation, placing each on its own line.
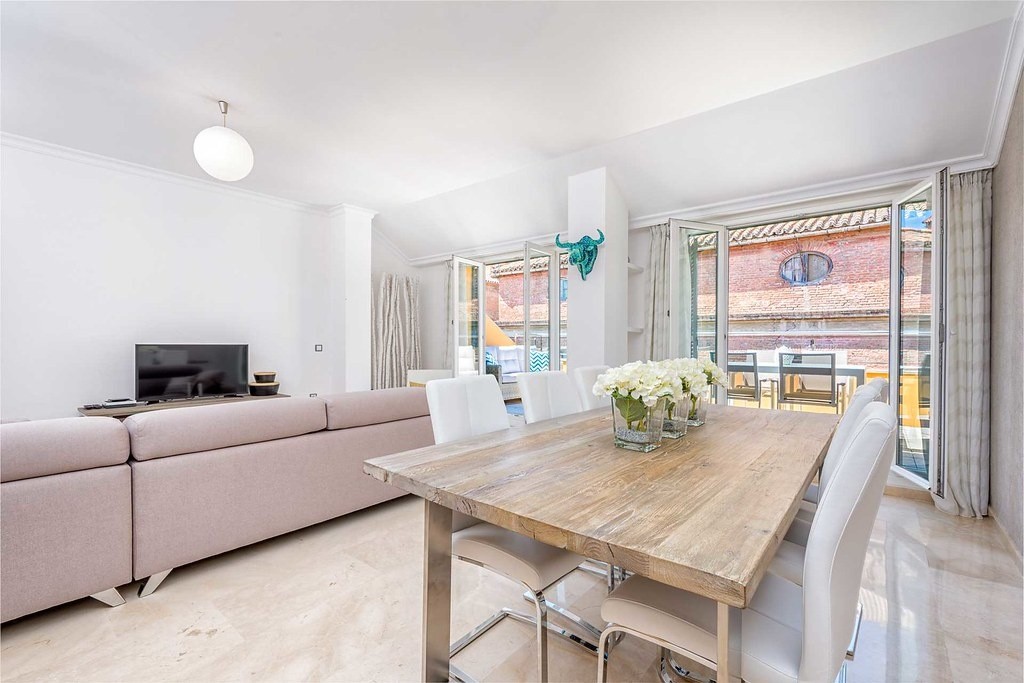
[486, 352, 498, 365]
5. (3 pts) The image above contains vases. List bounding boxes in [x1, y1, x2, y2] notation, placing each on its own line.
[662, 391, 691, 439]
[687, 391, 709, 427]
[610, 397, 666, 453]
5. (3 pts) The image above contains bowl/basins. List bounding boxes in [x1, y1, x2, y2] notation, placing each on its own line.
[254, 372, 276, 383]
[248, 381, 280, 396]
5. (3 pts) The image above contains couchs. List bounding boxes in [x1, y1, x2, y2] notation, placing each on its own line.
[0, 415, 132, 624]
[474, 344, 539, 401]
[122, 387, 436, 598]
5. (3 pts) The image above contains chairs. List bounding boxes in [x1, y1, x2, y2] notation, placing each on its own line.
[777, 352, 846, 414]
[425, 369, 632, 683]
[710, 352, 774, 408]
[596, 379, 898, 683]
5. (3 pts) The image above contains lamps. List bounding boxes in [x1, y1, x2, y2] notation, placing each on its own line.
[192, 100, 255, 182]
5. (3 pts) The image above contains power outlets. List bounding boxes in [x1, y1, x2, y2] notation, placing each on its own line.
[309, 393, 317, 397]
[315, 345, 322, 352]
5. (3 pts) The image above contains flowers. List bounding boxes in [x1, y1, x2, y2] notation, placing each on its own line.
[592, 359, 676, 407]
[660, 357, 709, 400]
[697, 355, 729, 389]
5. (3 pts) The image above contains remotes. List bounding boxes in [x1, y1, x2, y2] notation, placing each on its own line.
[84, 404, 102, 410]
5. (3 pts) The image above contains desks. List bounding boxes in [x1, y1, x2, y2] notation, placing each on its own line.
[729, 363, 865, 386]
[77, 387, 291, 422]
[362, 401, 842, 683]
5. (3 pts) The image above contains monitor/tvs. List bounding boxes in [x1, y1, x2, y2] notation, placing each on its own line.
[134, 344, 249, 406]
